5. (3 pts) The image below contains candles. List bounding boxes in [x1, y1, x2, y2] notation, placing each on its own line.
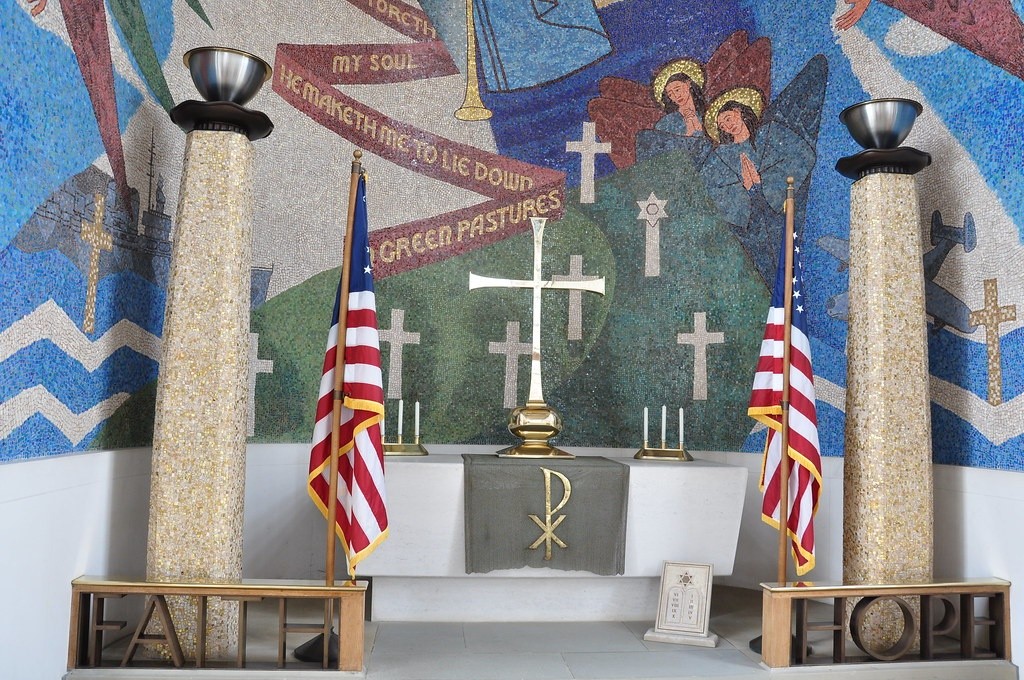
[679, 406, 685, 441]
[661, 404, 667, 441]
[414, 400, 420, 436]
[398, 399, 404, 434]
[380, 400, 386, 435]
[644, 406, 649, 441]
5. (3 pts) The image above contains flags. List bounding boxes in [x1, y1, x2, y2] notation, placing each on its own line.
[306, 170, 389, 582]
[746, 200, 822, 577]
